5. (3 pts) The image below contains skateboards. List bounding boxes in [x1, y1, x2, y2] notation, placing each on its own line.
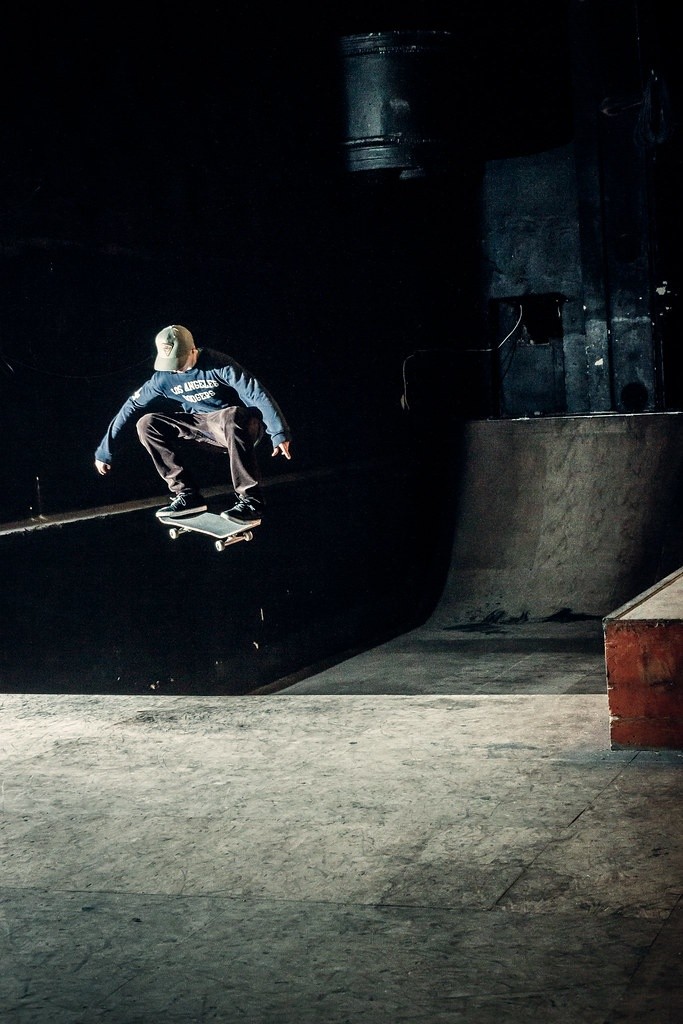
[158, 510, 261, 553]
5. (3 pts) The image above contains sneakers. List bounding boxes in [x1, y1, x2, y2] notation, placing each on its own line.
[219, 499, 262, 525]
[155, 491, 207, 519]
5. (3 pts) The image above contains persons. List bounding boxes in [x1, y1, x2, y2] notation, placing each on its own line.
[94, 325, 294, 528]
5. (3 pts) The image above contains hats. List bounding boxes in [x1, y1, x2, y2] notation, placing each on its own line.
[153, 324, 194, 372]
